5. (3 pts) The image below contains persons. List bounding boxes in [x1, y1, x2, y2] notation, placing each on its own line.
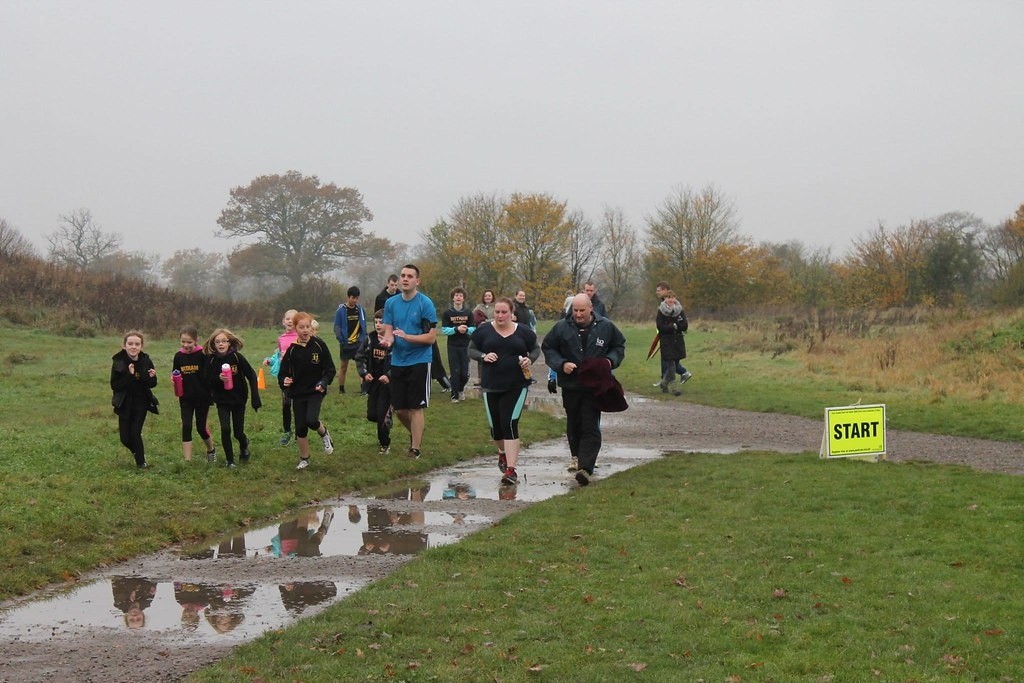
[513, 290, 537, 332]
[263, 310, 336, 469]
[334, 286, 368, 395]
[548, 282, 607, 394]
[172, 328, 262, 469]
[655, 282, 693, 395]
[111, 481, 517, 632]
[110, 332, 158, 469]
[542, 293, 626, 486]
[356, 274, 402, 455]
[442, 287, 476, 403]
[467, 297, 540, 484]
[474, 290, 495, 385]
[431, 339, 452, 392]
[380, 265, 439, 460]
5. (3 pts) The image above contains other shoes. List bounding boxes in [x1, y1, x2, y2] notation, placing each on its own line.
[660, 381, 669, 393]
[408, 446, 421, 459]
[548, 379, 557, 394]
[575, 468, 590, 484]
[673, 390, 681, 396]
[451, 399, 459, 402]
[226, 461, 235, 469]
[498, 453, 507, 473]
[442, 388, 452, 393]
[281, 430, 292, 446]
[207, 447, 216, 462]
[501, 466, 517, 484]
[568, 456, 579, 471]
[379, 444, 390, 455]
[321, 431, 334, 455]
[239, 438, 249, 459]
[138, 462, 146, 468]
[653, 381, 661, 386]
[296, 460, 310, 470]
[679, 371, 692, 384]
[458, 391, 465, 400]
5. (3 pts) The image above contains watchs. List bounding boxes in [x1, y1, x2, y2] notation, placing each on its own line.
[481, 353, 486, 360]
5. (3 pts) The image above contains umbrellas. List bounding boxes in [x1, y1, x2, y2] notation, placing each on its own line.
[646, 333, 660, 362]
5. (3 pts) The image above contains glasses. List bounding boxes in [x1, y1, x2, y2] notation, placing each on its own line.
[214, 338, 229, 345]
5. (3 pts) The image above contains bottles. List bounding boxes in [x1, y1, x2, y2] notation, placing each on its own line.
[519, 356, 533, 380]
[173, 369, 183, 397]
[222, 363, 233, 391]
